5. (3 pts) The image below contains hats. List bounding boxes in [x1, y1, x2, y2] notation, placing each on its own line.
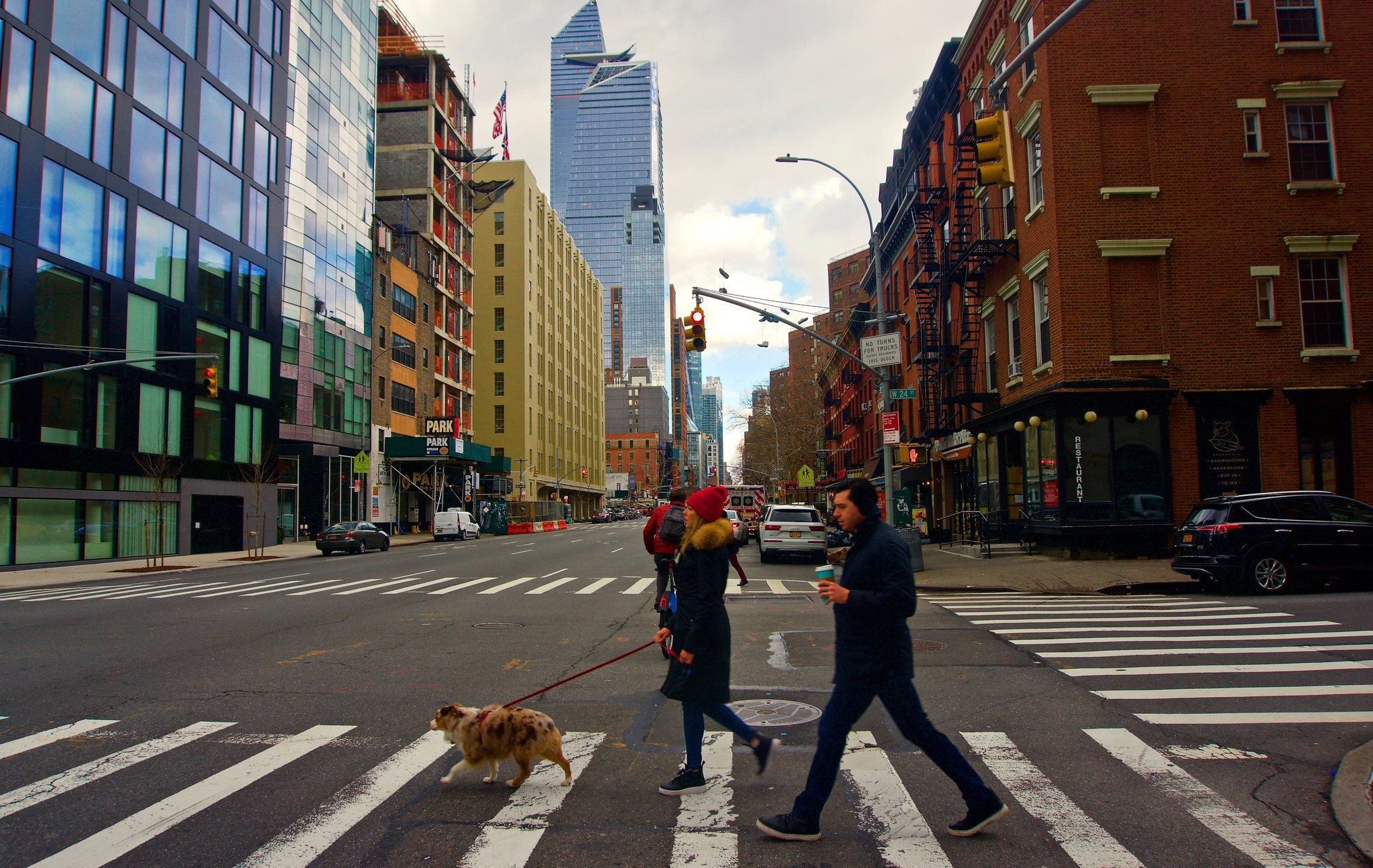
[686, 486, 727, 523]
[669, 486, 687, 502]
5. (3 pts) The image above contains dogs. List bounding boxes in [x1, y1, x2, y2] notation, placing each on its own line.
[428, 703, 572, 787]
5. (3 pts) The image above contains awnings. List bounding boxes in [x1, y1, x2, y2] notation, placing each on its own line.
[860, 458, 881, 480]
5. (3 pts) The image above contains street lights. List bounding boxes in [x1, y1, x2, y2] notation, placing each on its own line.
[748, 406, 782, 505]
[557, 427, 580, 501]
[628, 465, 646, 508]
[670, 477, 679, 492]
[772, 152, 895, 530]
[359, 345, 410, 521]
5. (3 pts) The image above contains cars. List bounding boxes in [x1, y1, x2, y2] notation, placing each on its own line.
[1069, 494, 1165, 524]
[315, 521, 390, 557]
[755, 502, 828, 564]
[591, 507, 643, 523]
[724, 510, 750, 547]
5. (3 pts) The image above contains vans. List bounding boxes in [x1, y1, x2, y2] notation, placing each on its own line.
[432, 507, 481, 542]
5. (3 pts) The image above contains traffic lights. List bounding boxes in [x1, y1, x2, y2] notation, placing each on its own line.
[203, 367, 218, 398]
[533, 468, 539, 477]
[683, 307, 707, 353]
[713, 468, 717, 477]
[550, 494, 555, 499]
[671, 487, 672, 490]
[894, 444, 928, 465]
[634, 491, 637, 494]
[582, 470, 588, 478]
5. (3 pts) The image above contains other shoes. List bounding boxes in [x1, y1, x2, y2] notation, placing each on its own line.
[737, 580, 749, 586]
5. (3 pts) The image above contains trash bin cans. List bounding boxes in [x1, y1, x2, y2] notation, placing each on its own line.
[897, 526, 925, 573]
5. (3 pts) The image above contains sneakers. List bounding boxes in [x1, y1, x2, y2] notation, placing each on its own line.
[948, 787, 1008, 837]
[756, 810, 821, 842]
[659, 760, 708, 796]
[741, 730, 780, 779]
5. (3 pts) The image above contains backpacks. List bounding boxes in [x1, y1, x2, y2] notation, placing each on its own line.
[656, 504, 686, 544]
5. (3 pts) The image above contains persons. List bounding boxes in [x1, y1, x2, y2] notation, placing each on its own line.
[756, 478, 1009, 841]
[652, 486, 782, 796]
[721, 510, 749, 586]
[564, 510, 568, 520]
[643, 486, 687, 610]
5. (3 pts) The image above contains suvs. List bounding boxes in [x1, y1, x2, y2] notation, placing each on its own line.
[1169, 489, 1373, 597]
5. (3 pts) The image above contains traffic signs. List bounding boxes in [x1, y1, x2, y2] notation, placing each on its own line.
[515, 481, 526, 489]
[769, 467, 784, 481]
[554, 486, 562, 489]
[875, 395, 884, 414]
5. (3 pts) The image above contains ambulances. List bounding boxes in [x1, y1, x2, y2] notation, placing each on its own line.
[719, 483, 767, 537]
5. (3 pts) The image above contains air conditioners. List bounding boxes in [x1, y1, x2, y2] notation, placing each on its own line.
[823, 408, 826, 414]
[868, 400, 872, 407]
[861, 403, 868, 411]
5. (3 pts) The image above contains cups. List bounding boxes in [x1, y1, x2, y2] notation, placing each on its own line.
[815, 564, 834, 603]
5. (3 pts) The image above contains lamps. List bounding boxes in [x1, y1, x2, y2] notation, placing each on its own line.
[1083, 410, 1098, 423]
[977, 432, 988, 442]
[1029, 416, 1042, 427]
[1012, 419, 1027, 431]
[967, 432, 976, 445]
[1134, 408, 1149, 422]
